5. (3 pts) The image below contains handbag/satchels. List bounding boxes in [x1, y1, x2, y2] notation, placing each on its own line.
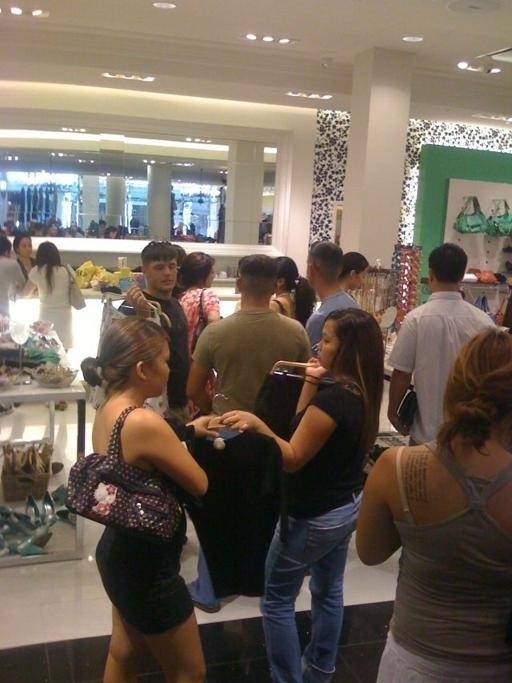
[487, 198, 512, 238]
[191, 287, 224, 355]
[64, 265, 86, 310]
[63, 403, 188, 550]
[453, 196, 490, 236]
[472, 293, 510, 328]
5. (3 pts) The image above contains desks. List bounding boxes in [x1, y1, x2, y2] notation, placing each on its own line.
[1, 364, 91, 572]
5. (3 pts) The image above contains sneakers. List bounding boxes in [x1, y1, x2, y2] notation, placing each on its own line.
[186, 584, 220, 614]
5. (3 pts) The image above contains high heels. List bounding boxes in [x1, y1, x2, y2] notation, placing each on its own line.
[1, 483, 76, 556]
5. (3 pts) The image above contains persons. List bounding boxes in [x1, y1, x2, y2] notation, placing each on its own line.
[355, 327, 512, 683]
[387, 243, 496, 446]
